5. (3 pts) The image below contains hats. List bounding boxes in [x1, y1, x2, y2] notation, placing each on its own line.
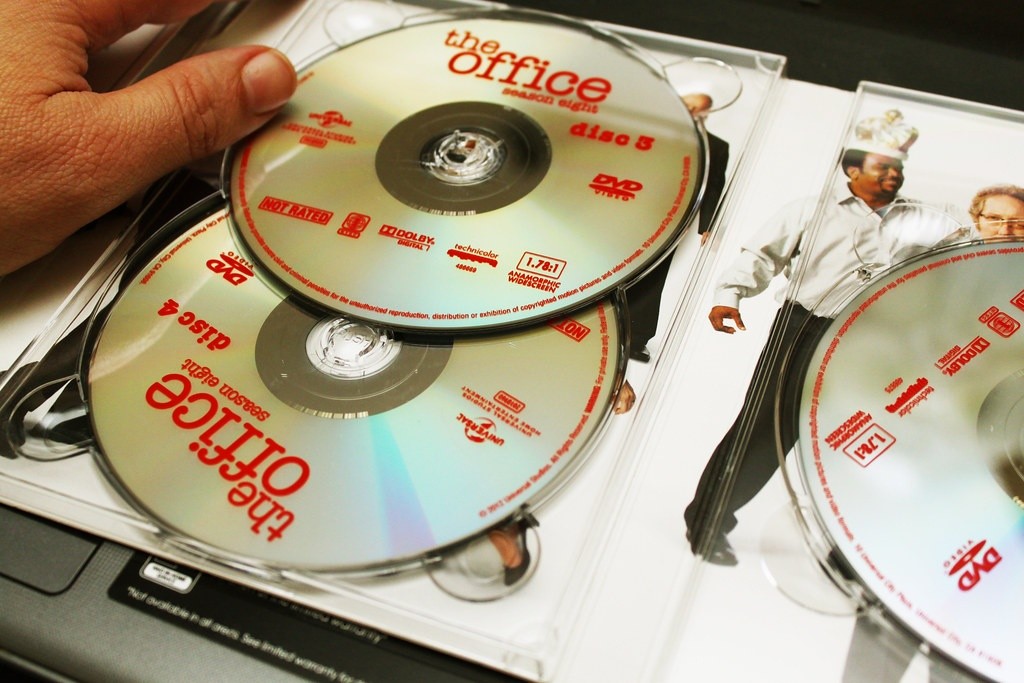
[843, 109, 919, 160]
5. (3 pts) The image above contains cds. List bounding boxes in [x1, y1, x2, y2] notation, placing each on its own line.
[86, 11, 709, 579]
[797, 238, 1024, 682]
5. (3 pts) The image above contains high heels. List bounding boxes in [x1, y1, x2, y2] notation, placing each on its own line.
[503, 514, 540, 586]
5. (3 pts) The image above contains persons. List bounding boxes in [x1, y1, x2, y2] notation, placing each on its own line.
[0, 0, 297, 273]
[489, 520, 531, 586]
[822, 547, 986, 682]
[970, 185, 1024, 244]
[617, 94, 730, 364]
[684, 108, 978, 567]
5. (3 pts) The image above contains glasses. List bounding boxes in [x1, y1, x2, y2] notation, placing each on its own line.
[979, 213, 1024, 230]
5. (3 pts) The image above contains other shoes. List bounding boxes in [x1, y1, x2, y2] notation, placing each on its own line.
[628, 345, 650, 361]
[686, 524, 739, 565]
